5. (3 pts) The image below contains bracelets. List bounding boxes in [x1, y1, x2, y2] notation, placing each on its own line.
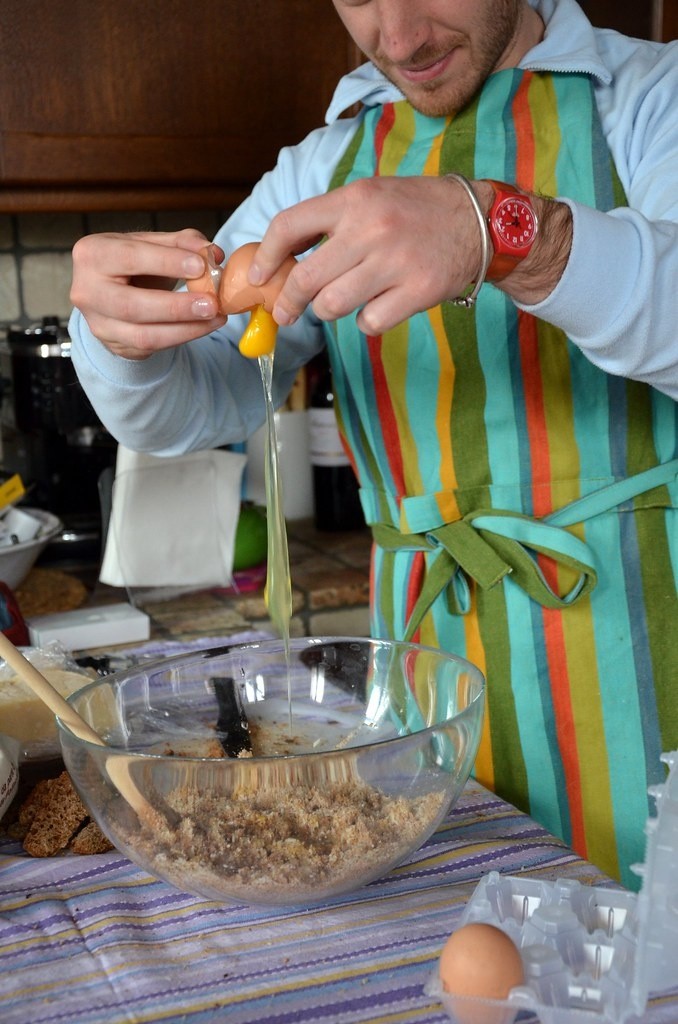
[436, 172, 488, 311]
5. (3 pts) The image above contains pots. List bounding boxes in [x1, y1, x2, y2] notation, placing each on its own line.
[8, 315, 99, 436]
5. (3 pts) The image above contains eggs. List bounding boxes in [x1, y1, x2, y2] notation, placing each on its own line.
[440, 923, 524, 1023]
[185, 242, 302, 735]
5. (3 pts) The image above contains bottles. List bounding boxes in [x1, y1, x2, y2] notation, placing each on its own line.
[306, 349, 371, 535]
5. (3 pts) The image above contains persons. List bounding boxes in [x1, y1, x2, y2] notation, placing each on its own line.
[69, 0, 676, 890]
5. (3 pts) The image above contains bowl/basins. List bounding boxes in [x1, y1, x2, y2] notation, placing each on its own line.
[0, 507, 65, 592]
[53, 636, 485, 905]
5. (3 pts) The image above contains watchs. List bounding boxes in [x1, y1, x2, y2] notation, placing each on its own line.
[474, 176, 540, 285]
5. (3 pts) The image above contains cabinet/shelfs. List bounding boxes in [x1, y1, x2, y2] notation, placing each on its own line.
[0, 0, 678, 212]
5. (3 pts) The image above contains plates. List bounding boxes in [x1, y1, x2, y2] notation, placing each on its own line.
[1, 670, 119, 759]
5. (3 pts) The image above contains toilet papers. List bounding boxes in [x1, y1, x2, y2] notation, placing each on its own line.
[237, 412, 314, 521]
